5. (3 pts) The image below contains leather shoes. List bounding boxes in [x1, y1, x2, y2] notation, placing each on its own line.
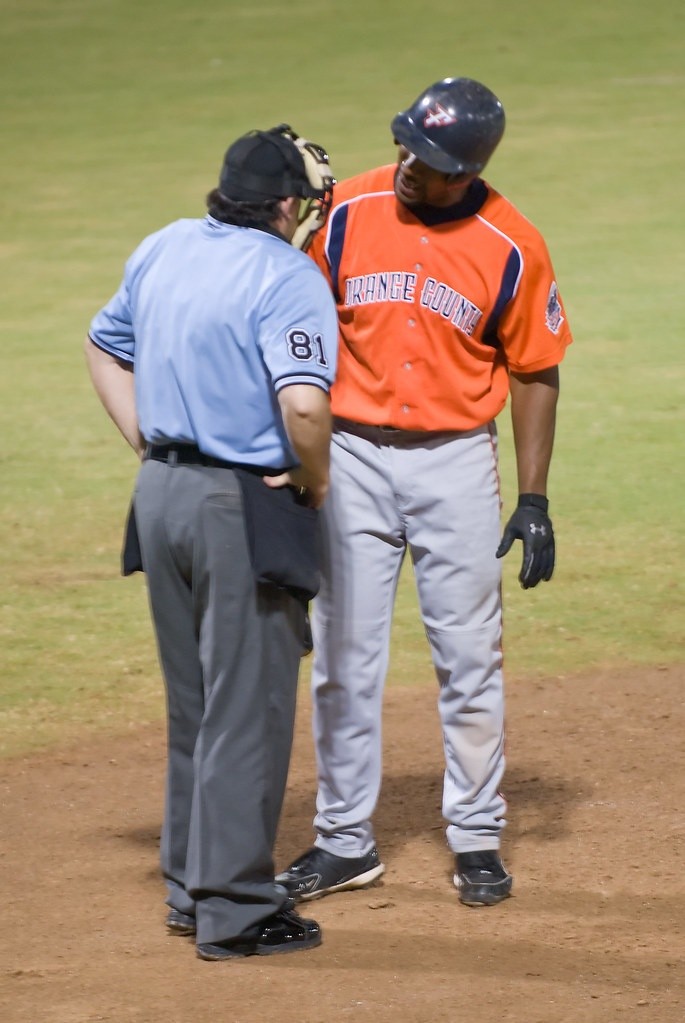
[198, 909, 322, 961]
[166, 909, 196, 933]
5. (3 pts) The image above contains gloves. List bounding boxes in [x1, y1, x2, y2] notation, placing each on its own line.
[496, 493, 556, 589]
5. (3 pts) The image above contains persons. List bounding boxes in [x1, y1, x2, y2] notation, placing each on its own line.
[276, 76, 575, 907]
[86, 125, 340, 960]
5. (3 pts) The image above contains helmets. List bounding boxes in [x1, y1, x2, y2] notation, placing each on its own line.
[391, 77, 505, 184]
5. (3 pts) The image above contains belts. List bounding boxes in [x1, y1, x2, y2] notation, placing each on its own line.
[357, 424, 399, 433]
[148, 445, 232, 469]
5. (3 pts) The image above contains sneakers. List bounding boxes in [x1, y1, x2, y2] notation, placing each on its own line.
[453, 848, 512, 907]
[275, 846, 385, 901]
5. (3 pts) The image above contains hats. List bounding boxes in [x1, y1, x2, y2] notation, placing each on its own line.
[219, 130, 320, 204]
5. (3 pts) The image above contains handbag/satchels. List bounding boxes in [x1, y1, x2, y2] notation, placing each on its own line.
[235, 469, 325, 600]
